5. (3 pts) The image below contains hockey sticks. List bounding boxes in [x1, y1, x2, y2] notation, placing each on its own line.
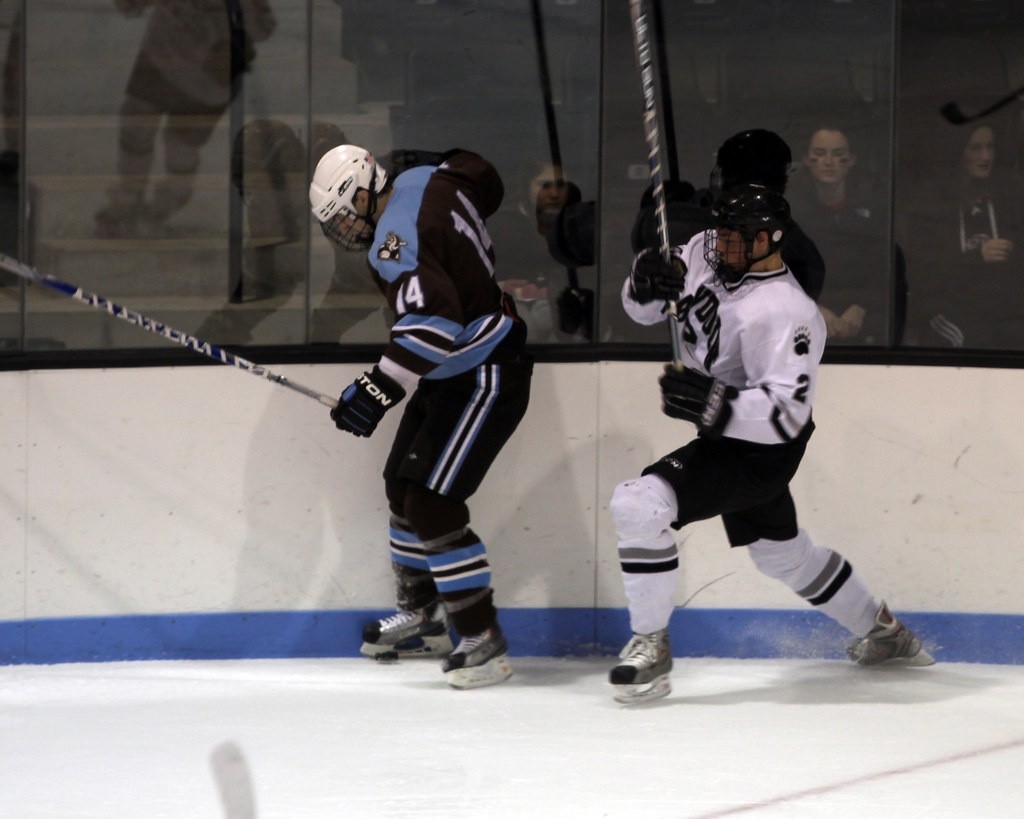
[621, 0, 689, 375]
[0, 253, 340, 410]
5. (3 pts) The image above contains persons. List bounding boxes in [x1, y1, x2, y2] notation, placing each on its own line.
[93, 2, 386, 343]
[603, 178, 939, 713]
[630, 128, 828, 300]
[896, 115, 1024, 352]
[309, 146, 535, 688]
[787, 122, 898, 353]
[477, 149, 613, 349]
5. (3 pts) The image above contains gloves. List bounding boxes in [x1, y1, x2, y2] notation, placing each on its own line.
[329, 363, 408, 438]
[658, 362, 741, 436]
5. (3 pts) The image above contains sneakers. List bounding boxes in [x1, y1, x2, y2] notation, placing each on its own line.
[844, 599, 938, 667]
[629, 245, 688, 305]
[359, 597, 454, 662]
[443, 620, 514, 691]
[608, 624, 673, 705]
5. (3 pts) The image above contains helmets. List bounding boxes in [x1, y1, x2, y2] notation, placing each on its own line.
[308, 143, 388, 224]
[709, 183, 790, 263]
